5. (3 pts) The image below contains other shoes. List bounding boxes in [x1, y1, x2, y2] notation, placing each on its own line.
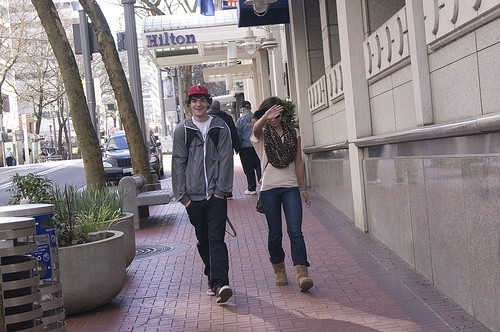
[226, 192, 233, 199]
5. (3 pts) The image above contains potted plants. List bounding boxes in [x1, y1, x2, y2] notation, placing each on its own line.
[5, 171, 135, 315]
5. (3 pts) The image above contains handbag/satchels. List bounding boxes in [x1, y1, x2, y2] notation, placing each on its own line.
[256, 193, 266, 214]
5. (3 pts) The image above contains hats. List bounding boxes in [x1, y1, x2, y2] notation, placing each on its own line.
[188, 85, 208, 95]
[240, 101, 250, 108]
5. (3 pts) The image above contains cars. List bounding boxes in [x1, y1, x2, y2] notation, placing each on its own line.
[102, 130, 164, 180]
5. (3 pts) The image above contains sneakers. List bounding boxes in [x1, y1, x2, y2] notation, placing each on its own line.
[244, 188, 256, 195]
[207, 288, 216, 296]
[216, 285, 232, 303]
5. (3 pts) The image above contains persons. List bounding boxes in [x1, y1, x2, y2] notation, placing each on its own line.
[207, 99, 241, 198]
[235, 101, 263, 195]
[40, 144, 68, 161]
[250, 96, 313, 292]
[171, 86, 232, 303]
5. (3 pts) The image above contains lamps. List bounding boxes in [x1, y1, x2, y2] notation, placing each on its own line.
[243, 0, 276, 17]
[240, 25, 284, 54]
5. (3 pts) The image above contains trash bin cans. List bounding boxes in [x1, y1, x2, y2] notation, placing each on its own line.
[0, 216, 46, 332]
[0, 204, 68, 332]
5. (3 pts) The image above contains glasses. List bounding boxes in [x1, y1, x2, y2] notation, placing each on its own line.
[275, 115, 280, 119]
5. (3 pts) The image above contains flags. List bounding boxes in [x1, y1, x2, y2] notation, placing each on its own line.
[195, 0, 215, 16]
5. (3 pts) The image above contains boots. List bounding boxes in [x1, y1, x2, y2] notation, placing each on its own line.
[273, 260, 288, 285]
[294, 264, 313, 291]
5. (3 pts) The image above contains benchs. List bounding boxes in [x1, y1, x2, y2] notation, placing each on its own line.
[118, 174, 174, 230]
